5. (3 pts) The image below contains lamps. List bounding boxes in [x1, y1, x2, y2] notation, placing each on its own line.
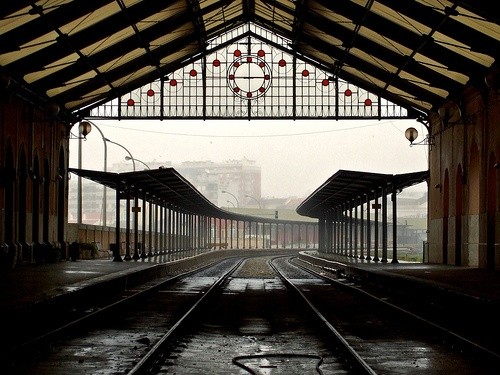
[405, 128, 436, 151]
[60, 121, 91, 140]
[484, 75, 500, 98]
[438, 105, 477, 128]
[23, 104, 61, 123]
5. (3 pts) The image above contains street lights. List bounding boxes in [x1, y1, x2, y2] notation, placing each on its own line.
[78, 118, 92, 139]
[406, 125, 430, 148]
[83, 119, 108, 227]
[104, 135, 137, 233]
